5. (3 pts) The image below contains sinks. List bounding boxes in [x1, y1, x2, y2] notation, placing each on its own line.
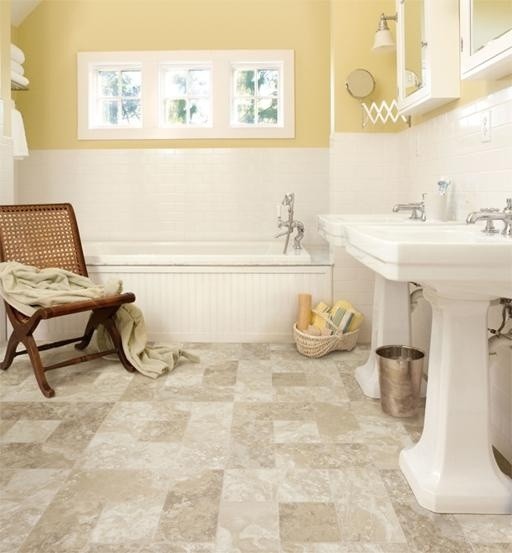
[345, 220, 512, 303]
[318, 214, 428, 246]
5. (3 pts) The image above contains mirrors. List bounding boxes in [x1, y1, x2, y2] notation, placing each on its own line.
[344, 67, 376, 98]
[396, 0, 430, 113]
[458, 0, 512, 74]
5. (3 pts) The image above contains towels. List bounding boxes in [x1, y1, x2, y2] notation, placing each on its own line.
[0, 259, 202, 379]
[9, 42, 31, 87]
[11, 106, 29, 161]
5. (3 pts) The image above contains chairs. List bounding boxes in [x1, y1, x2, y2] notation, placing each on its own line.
[0, 202, 137, 398]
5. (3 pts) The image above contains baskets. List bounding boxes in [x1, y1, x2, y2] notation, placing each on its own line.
[292, 306, 364, 361]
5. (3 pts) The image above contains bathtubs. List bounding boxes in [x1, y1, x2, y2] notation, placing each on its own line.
[82, 241, 333, 341]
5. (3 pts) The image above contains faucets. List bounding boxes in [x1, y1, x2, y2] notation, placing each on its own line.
[274, 229, 291, 240]
[392, 203, 428, 221]
[466, 198, 512, 233]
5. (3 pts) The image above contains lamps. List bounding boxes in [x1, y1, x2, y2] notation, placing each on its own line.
[371, 10, 398, 51]
